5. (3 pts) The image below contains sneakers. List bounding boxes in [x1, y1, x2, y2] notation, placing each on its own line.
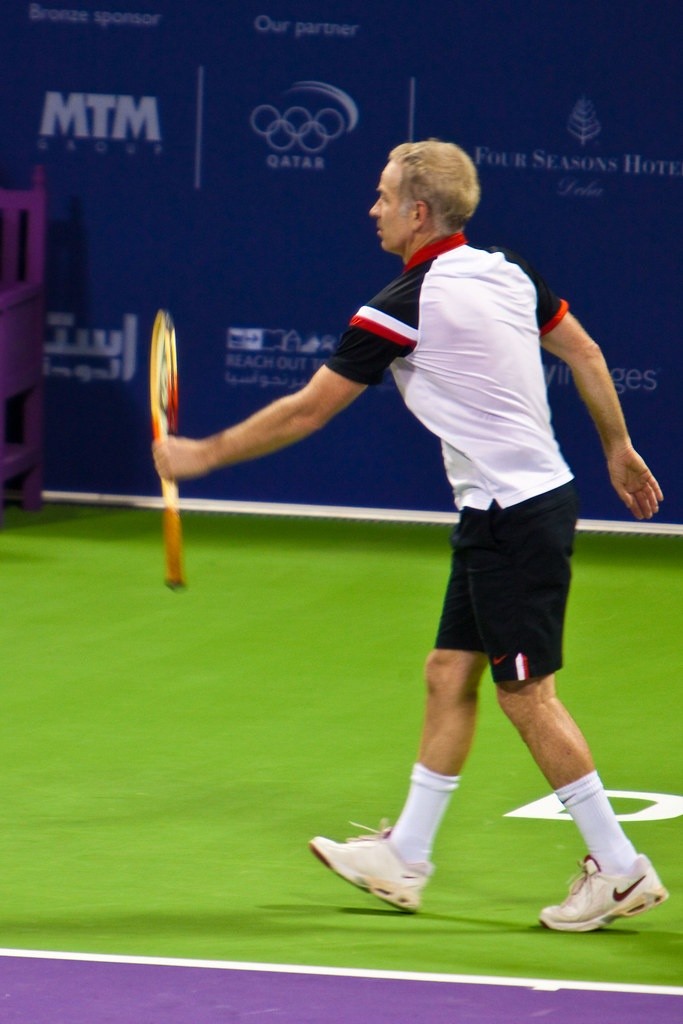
[308, 818, 431, 912]
[538, 854, 671, 932]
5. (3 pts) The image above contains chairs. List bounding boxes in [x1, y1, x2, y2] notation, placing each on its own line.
[0, 167, 49, 512]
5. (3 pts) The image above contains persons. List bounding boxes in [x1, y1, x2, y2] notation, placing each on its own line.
[153, 140, 670, 931]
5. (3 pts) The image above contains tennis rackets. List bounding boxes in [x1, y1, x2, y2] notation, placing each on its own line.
[150, 309, 188, 593]
